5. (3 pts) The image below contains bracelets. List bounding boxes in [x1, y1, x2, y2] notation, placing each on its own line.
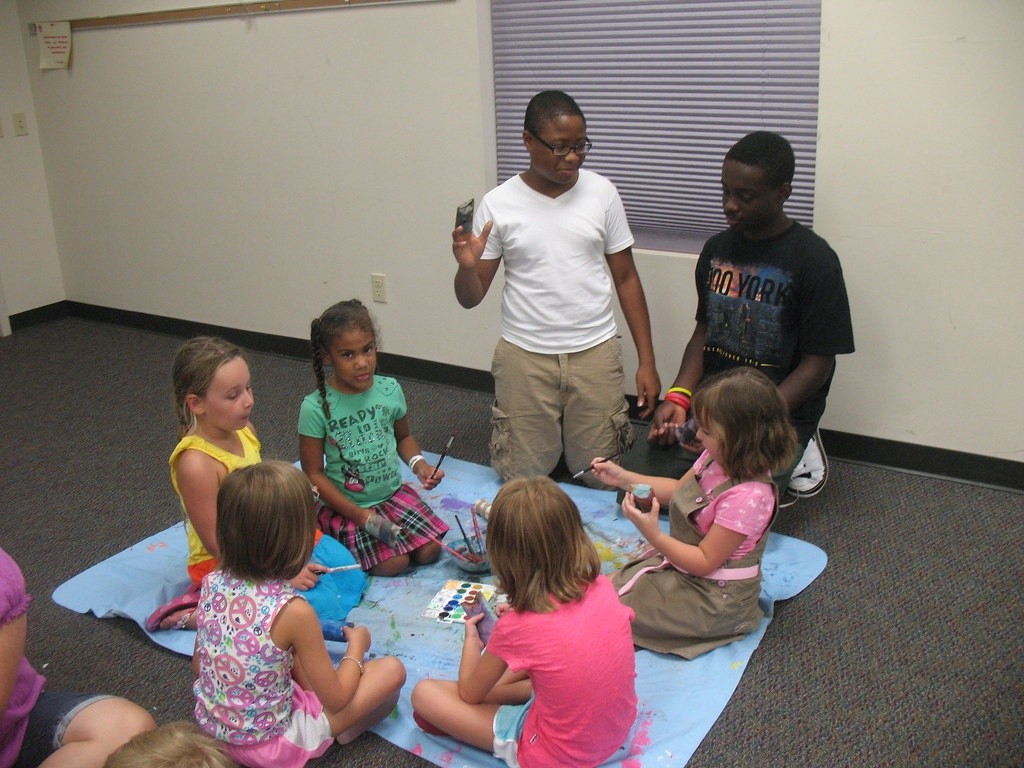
[664, 386, 692, 411]
[340, 656, 363, 674]
[409, 454, 423, 471]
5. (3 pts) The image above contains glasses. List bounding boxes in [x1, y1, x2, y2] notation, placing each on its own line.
[532, 133, 592, 157]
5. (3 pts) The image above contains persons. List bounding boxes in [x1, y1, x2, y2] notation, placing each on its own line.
[411, 475, 638, 768]
[646, 131, 855, 499]
[590, 366, 799, 661]
[191, 459, 407, 768]
[297, 298, 450, 577]
[104, 720, 239, 768]
[452, 91, 661, 491]
[0, 547, 158, 768]
[144, 335, 329, 633]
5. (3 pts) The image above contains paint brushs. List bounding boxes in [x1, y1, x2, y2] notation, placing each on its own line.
[470, 507, 485, 556]
[455, 515, 473, 554]
[567, 450, 626, 482]
[427, 534, 472, 562]
[430, 429, 459, 480]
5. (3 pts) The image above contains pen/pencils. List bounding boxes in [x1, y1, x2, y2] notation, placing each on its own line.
[314, 564, 360, 574]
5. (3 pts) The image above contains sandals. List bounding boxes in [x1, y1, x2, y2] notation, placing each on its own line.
[147, 584, 201, 632]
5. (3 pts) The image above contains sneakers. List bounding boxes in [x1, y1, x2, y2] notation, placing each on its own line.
[778, 430, 829, 509]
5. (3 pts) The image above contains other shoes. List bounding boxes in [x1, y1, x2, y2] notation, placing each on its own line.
[338, 690, 400, 745]
[412, 709, 450, 738]
